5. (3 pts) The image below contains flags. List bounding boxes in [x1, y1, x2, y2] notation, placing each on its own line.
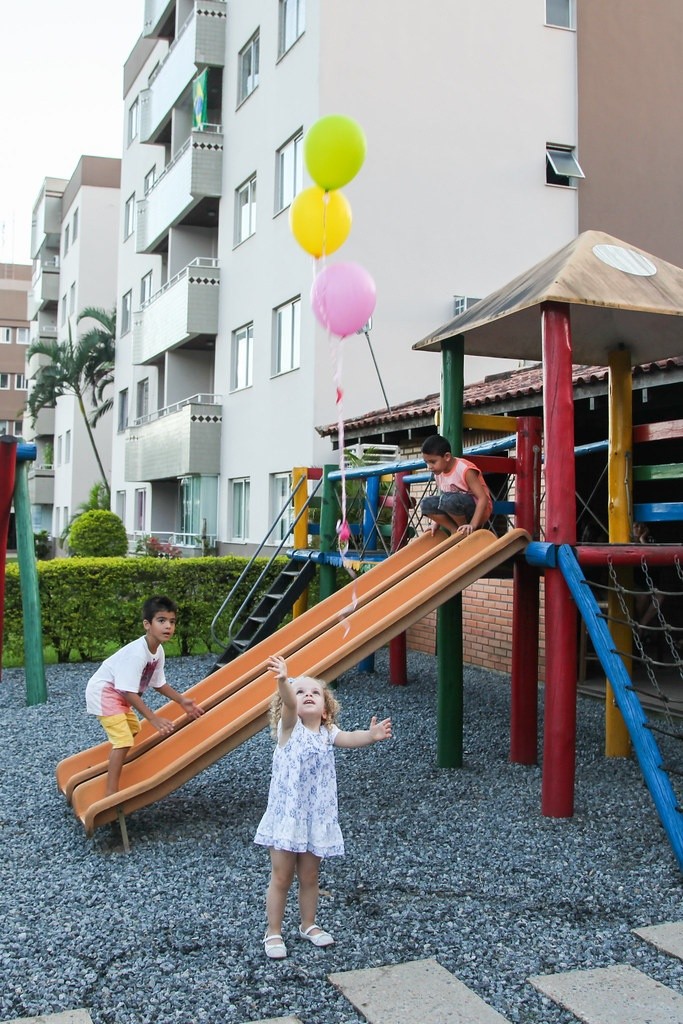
[190, 68, 209, 129]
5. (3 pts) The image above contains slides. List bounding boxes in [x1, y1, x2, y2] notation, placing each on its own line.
[56, 519, 535, 827]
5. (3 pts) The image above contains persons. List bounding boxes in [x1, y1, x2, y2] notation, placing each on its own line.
[580, 508, 667, 642]
[420, 435, 493, 537]
[84, 595, 204, 797]
[253, 655, 392, 959]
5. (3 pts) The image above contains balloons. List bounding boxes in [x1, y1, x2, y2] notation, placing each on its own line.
[312, 262, 377, 338]
[291, 187, 352, 257]
[303, 113, 366, 194]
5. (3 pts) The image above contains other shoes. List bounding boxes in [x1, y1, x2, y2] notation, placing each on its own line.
[264, 932, 287, 959]
[299, 924, 335, 947]
